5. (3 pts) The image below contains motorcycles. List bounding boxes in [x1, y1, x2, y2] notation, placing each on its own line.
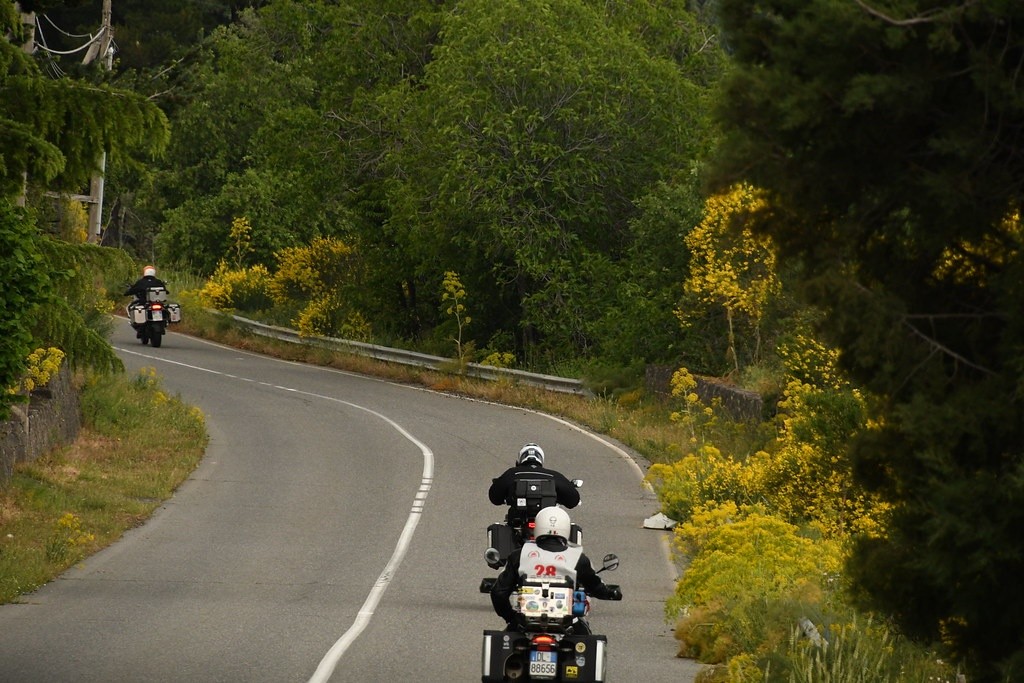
[125, 280, 171, 348]
[477, 547, 622, 683]
[485, 476, 584, 566]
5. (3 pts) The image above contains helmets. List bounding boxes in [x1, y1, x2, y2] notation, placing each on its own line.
[534, 506, 572, 544]
[516, 443, 546, 467]
[143, 265, 156, 278]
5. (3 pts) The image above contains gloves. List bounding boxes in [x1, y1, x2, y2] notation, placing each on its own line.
[594, 583, 624, 603]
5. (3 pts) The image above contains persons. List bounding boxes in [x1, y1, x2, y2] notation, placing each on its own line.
[489, 441, 621, 634]
[124, 265, 169, 305]
